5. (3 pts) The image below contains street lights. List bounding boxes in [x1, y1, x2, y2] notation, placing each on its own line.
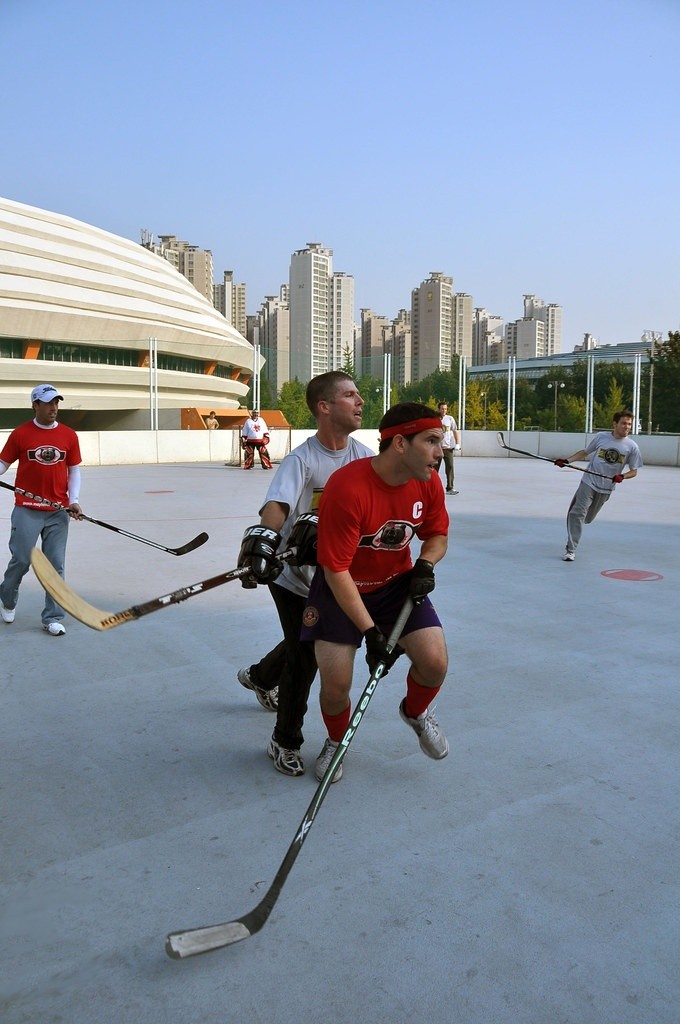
[642, 328, 666, 435]
[547, 380, 565, 432]
[481, 391, 487, 430]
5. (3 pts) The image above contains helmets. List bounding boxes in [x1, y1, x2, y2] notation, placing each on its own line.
[252, 409, 259, 418]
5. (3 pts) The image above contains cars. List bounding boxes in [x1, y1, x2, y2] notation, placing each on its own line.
[524, 426, 548, 432]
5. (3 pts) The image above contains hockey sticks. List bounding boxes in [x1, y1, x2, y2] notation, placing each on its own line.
[163, 600, 415, 962]
[497, 431, 613, 481]
[377, 437, 461, 451]
[28, 544, 296, 634]
[0, 481, 210, 557]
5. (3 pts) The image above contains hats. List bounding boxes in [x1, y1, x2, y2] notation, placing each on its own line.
[31, 384, 64, 403]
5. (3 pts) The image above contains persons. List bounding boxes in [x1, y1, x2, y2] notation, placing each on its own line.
[241, 409, 272, 470]
[554, 410, 643, 561]
[205, 411, 219, 430]
[0, 384, 84, 636]
[237, 371, 379, 777]
[434, 402, 460, 495]
[303, 402, 449, 783]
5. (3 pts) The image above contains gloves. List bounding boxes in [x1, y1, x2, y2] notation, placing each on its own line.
[612, 475, 624, 484]
[404, 558, 435, 608]
[364, 625, 405, 678]
[554, 458, 569, 468]
[285, 512, 319, 567]
[454, 444, 462, 452]
[237, 525, 285, 590]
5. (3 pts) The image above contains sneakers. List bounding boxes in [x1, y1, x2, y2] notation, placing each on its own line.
[314, 737, 349, 784]
[237, 666, 279, 711]
[399, 696, 449, 760]
[266, 733, 305, 777]
[562, 552, 575, 561]
[42, 622, 66, 636]
[0, 598, 15, 623]
[446, 489, 459, 495]
